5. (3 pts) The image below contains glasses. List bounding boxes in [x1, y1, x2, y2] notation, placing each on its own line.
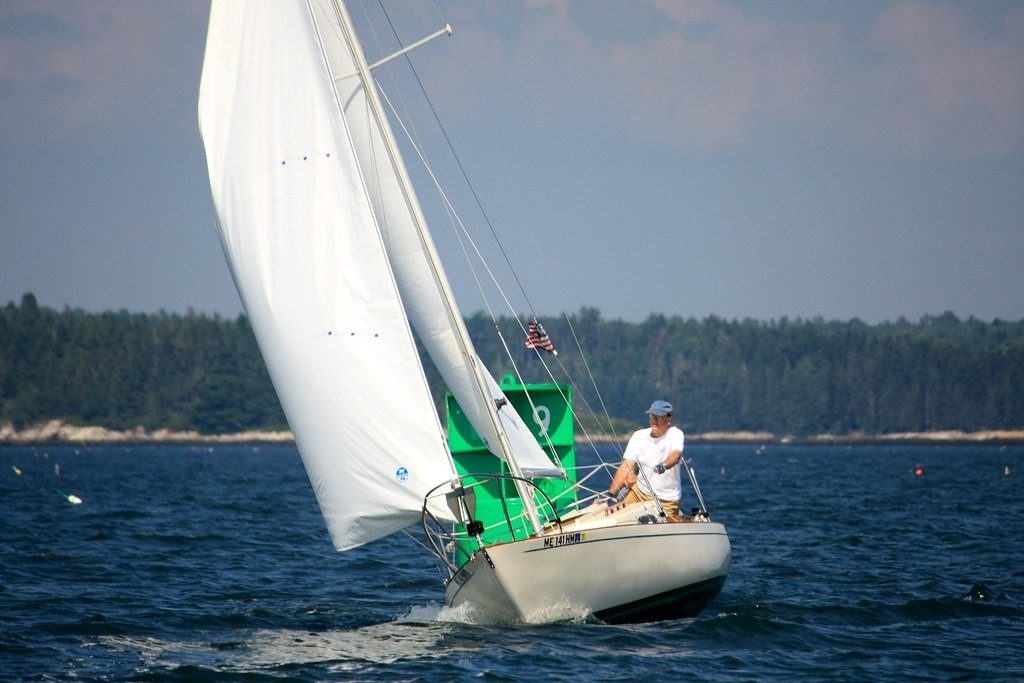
[649, 413, 666, 419]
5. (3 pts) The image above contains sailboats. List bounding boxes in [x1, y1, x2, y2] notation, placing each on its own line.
[198, 0, 732, 629]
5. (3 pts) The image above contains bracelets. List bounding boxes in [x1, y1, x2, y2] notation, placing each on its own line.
[662, 463, 667, 465]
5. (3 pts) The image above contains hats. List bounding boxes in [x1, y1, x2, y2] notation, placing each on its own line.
[645, 400, 673, 416]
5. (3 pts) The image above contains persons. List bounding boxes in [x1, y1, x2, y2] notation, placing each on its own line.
[592, 400, 684, 523]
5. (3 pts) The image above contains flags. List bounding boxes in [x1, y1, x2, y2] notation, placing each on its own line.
[524, 315, 556, 354]
